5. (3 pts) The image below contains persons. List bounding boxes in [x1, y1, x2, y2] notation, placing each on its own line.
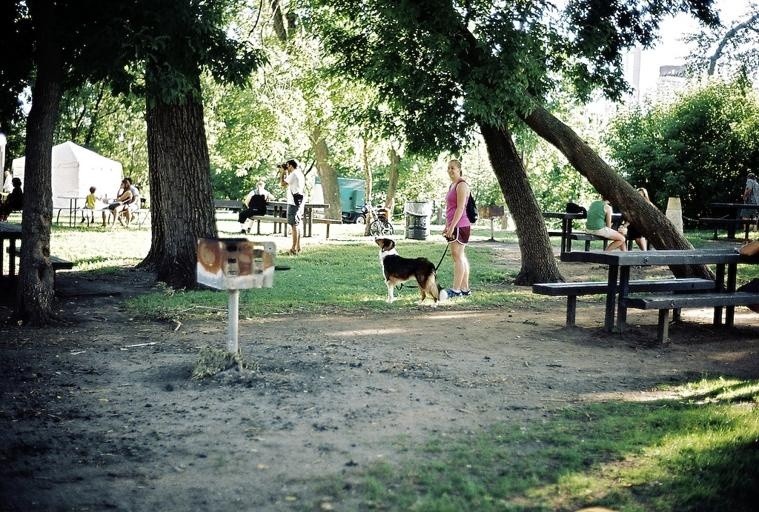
[618, 187, 651, 252]
[280, 159, 306, 254]
[740, 173, 758, 231]
[0, 194, 2, 205]
[108, 177, 140, 228]
[733, 236, 759, 315]
[237, 180, 276, 234]
[1, 177, 24, 222]
[102, 193, 109, 205]
[443, 159, 473, 296]
[585, 199, 626, 251]
[81, 186, 102, 223]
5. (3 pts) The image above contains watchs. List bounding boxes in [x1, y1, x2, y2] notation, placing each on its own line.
[97, 178, 133, 228]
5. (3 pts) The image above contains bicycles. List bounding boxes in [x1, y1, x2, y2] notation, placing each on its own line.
[359, 199, 396, 236]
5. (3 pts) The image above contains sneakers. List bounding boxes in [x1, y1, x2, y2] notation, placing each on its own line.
[444, 288, 462, 298]
[461, 289, 471, 296]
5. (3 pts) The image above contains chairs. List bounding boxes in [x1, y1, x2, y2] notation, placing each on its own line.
[53, 194, 149, 231]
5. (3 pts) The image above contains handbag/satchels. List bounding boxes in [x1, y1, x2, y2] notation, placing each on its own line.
[566, 203, 586, 218]
[292, 194, 303, 206]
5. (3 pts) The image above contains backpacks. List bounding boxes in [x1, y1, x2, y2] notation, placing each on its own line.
[456, 180, 478, 223]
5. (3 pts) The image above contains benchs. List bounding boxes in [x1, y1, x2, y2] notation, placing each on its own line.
[548, 230, 585, 242]
[698, 217, 757, 241]
[532, 278, 713, 325]
[250, 215, 288, 237]
[570, 230, 612, 251]
[6, 247, 74, 270]
[623, 292, 759, 346]
[300, 216, 342, 238]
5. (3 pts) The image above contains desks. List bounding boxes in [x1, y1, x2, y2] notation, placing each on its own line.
[543, 210, 622, 253]
[1, 220, 21, 240]
[712, 203, 759, 217]
[562, 247, 745, 328]
[266, 200, 330, 237]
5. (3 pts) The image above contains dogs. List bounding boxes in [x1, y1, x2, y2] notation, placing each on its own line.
[373, 236, 443, 310]
